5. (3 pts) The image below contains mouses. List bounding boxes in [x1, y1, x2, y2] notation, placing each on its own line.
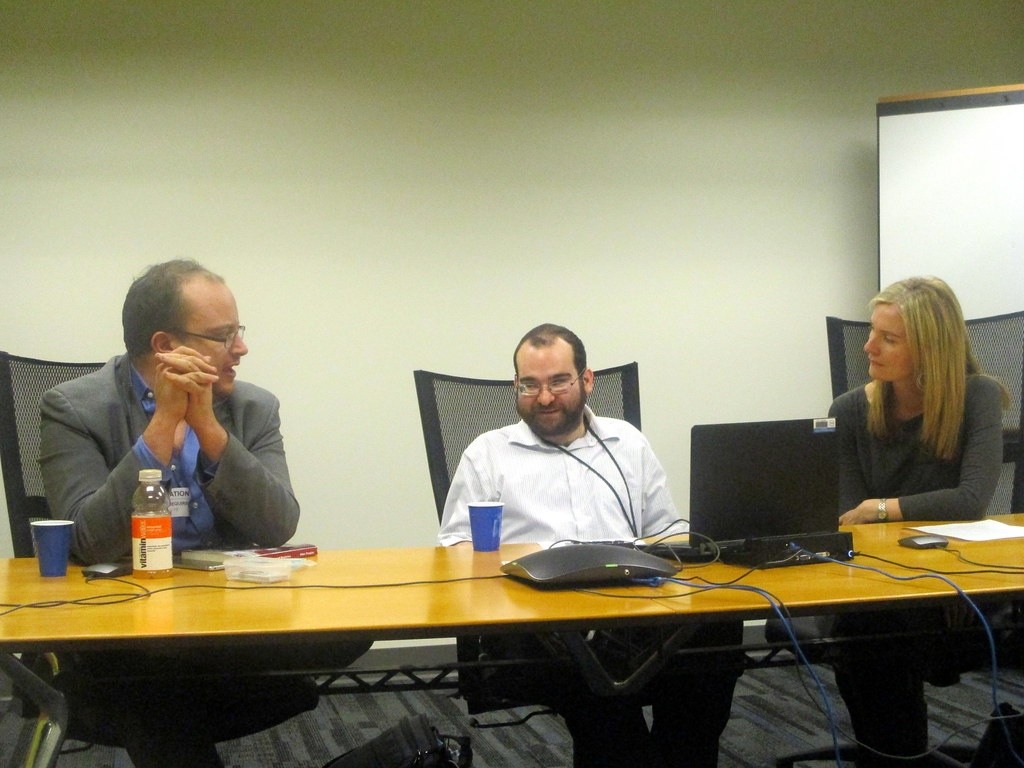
[897, 535, 949, 549]
[81, 562, 132, 578]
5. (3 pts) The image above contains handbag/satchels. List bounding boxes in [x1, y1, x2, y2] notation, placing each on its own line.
[323, 713, 473, 768]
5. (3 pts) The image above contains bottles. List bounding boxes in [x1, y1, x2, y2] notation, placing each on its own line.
[131, 469, 174, 579]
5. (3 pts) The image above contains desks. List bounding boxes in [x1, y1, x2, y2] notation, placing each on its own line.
[0, 513, 1024, 768]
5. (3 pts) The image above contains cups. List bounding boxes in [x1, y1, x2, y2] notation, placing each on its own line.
[30, 519, 74, 578]
[467, 502, 505, 552]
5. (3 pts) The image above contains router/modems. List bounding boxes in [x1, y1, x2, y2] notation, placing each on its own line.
[501, 543, 676, 584]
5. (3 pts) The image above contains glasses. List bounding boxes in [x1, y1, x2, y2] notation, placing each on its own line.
[518, 367, 586, 396]
[185, 325, 246, 349]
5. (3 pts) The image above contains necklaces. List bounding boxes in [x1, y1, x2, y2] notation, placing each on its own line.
[543, 424, 639, 539]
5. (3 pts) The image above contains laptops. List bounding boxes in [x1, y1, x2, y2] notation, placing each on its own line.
[632, 415, 841, 559]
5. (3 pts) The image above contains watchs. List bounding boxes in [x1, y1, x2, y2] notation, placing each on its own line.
[879, 499, 888, 522]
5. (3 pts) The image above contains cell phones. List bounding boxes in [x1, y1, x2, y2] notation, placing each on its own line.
[173, 558, 226, 571]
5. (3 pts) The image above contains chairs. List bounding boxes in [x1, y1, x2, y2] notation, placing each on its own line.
[413, 362, 653, 729]
[1, 352, 319, 746]
[765, 311, 1024, 686]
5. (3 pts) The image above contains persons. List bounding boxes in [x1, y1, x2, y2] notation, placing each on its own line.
[38, 259, 303, 768]
[827, 281, 1009, 768]
[432, 324, 745, 768]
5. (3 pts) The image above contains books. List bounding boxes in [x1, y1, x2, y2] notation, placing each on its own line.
[174, 545, 317, 572]
